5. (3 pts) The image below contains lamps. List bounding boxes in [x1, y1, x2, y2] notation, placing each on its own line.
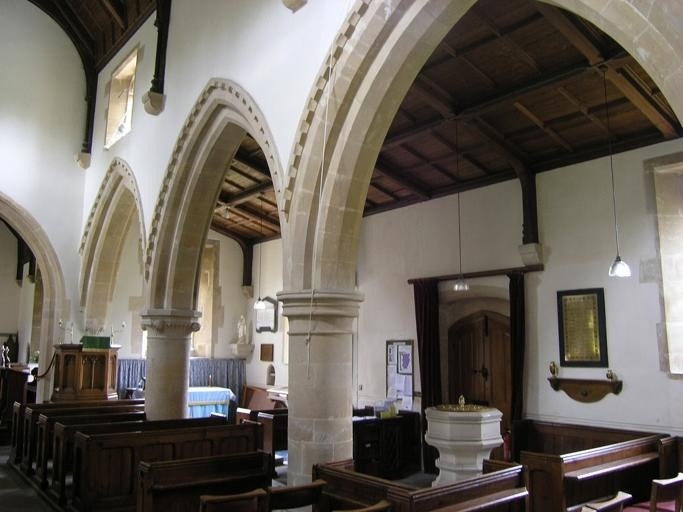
[448, 109, 470, 293]
[253, 189, 268, 312]
[596, 62, 633, 279]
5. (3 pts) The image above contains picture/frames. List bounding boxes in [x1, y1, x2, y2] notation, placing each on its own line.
[555, 287, 609, 370]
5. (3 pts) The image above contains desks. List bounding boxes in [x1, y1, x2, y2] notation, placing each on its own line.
[188, 387, 232, 419]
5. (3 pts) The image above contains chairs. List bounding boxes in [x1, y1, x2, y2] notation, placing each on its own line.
[198, 476, 390, 511]
[581, 470, 683, 512]
